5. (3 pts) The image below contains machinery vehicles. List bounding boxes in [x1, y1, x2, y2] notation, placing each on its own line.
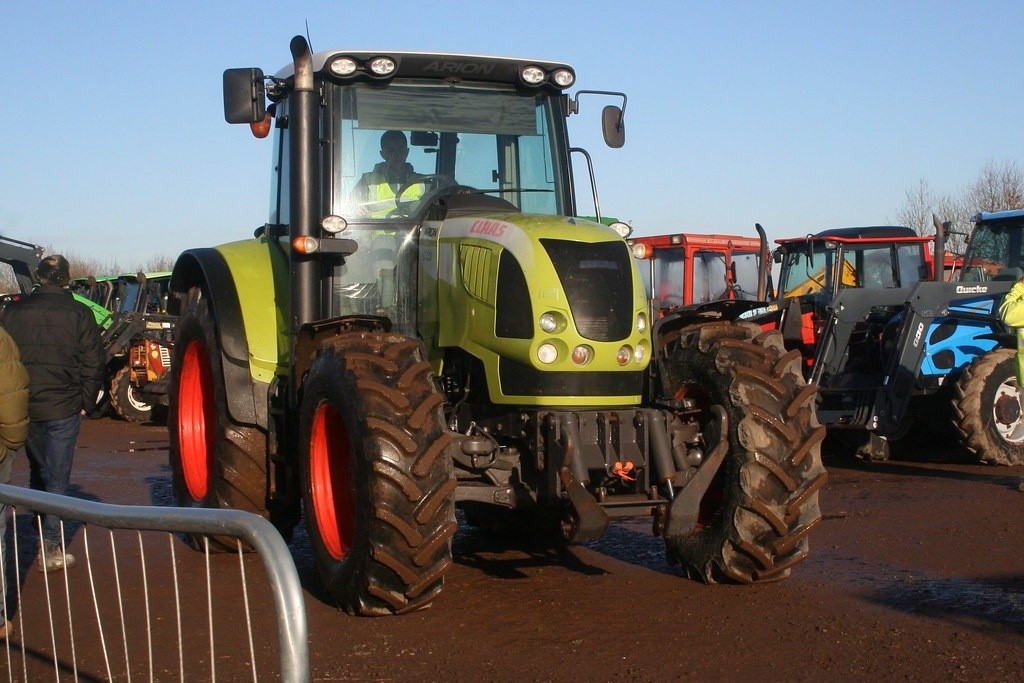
[619, 205, 1024, 468]
[159, 17, 828, 617]
[0, 237, 185, 427]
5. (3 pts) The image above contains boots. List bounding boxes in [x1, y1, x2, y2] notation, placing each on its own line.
[37, 536, 76, 573]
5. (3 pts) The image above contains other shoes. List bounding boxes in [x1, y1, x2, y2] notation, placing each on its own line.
[0, 619, 14, 639]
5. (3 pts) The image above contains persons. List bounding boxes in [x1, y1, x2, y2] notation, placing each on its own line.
[0, 325, 31, 642]
[0, 255, 106, 573]
[346, 130, 436, 282]
